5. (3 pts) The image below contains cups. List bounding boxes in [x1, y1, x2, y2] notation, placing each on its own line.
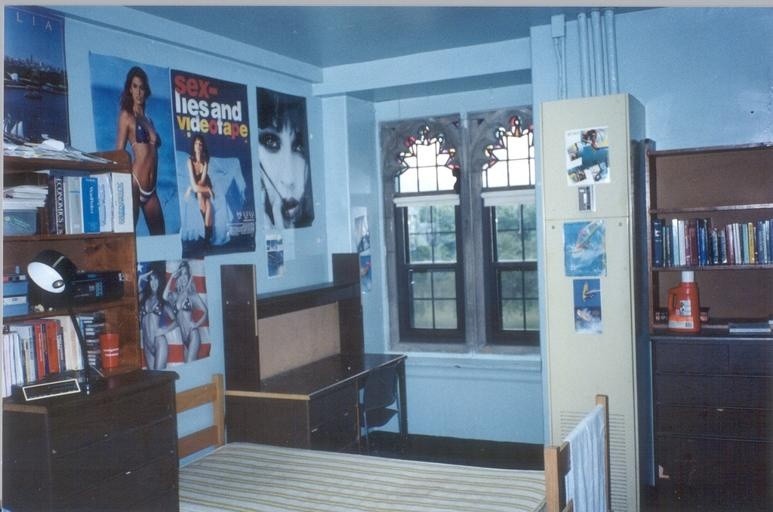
[99, 333, 120, 369]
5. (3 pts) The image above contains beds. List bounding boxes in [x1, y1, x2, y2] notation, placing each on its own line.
[177, 374, 613, 511]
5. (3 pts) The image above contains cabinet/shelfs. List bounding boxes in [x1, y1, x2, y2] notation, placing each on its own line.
[648, 141, 772, 511]
[536, 92, 647, 511]
[221, 253, 408, 447]
[3, 145, 180, 511]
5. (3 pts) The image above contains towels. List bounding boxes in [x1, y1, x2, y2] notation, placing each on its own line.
[565, 403, 607, 511]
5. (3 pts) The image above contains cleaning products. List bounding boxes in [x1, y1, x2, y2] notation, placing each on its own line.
[666, 271, 701, 333]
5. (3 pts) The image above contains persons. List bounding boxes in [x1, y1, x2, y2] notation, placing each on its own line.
[137, 261, 170, 372]
[163, 258, 208, 364]
[257, 86, 315, 230]
[117, 67, 166, 236]
[188, 135, 217, 249]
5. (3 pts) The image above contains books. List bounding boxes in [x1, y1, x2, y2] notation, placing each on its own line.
[1, 312, 113, 399]
[730, 324, 771, 335]
[647, 213, 773, 267]
[655, 308, 710, 322]
[2, 164, 132, 236]
